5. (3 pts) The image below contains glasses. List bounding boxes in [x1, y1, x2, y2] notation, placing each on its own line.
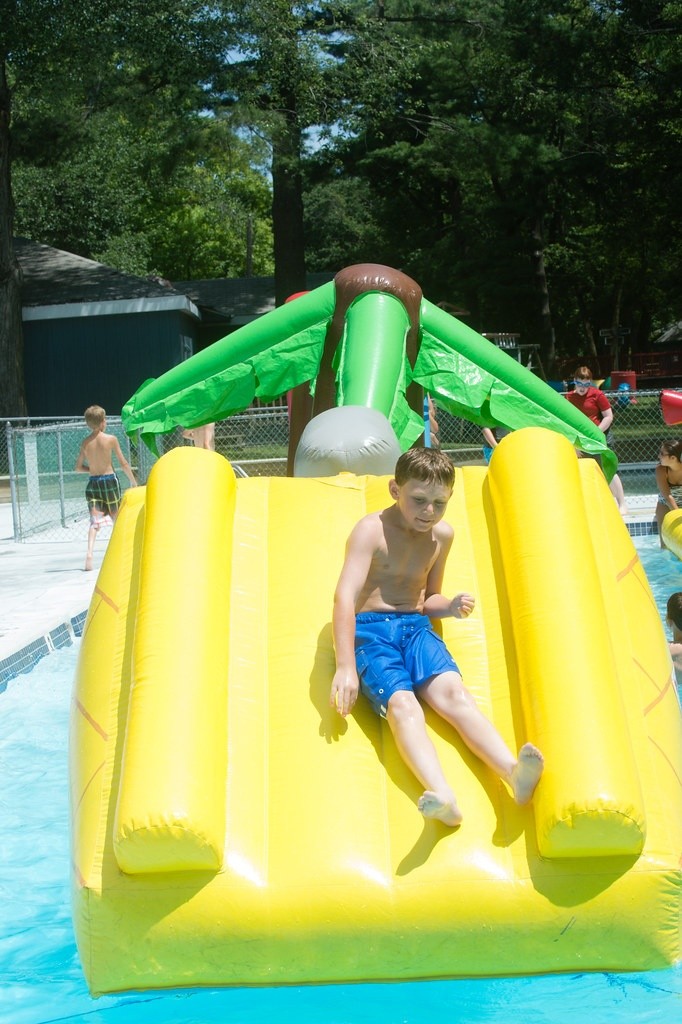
[571, 380, 591, 387]
[658, 449, 669, 458]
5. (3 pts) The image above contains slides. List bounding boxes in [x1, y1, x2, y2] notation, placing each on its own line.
[74, 426, 682, 992]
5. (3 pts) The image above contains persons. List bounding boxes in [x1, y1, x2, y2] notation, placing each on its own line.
[181, 422, 216, 453]
[77, 405, 139, 571]
[418, 364, 682, 673]
[333, 448, 544, 827]
